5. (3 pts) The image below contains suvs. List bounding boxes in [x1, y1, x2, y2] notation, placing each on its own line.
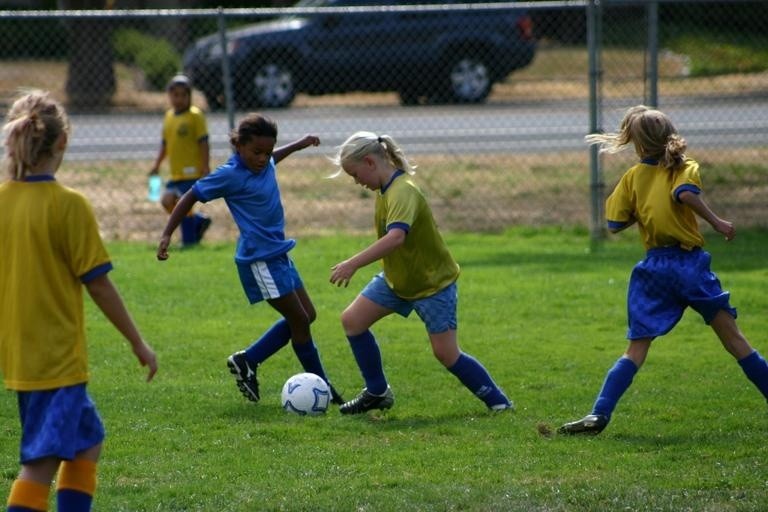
[183, 1, 535, 108]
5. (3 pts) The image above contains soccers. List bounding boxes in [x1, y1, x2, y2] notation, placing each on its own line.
[281, 372, 331, 416]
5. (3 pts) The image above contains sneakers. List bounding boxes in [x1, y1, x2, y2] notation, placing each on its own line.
[326, 381, 345, 405]
[340, 384, 395, 416]
[199, 216, 211, 239]
[228, 349, 259, 403]
[556, 414, 609, 436]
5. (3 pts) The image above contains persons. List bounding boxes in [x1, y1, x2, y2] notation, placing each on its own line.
[0, 90, 159, 512]
[326, 132, 516, 421]
[146, 74, 212, 252]
[156, 112, 345, 407]
[554, 105, 767, 436]
